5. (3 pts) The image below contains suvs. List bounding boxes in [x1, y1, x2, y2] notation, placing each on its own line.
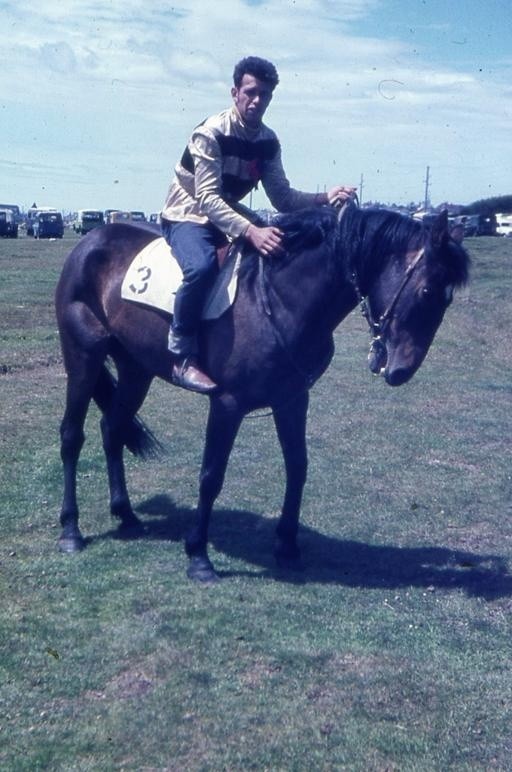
[0, 203, 164, 241]
[412, 210, 511, 238]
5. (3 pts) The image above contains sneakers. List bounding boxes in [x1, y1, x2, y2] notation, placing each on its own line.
[172, 361, 217, 391]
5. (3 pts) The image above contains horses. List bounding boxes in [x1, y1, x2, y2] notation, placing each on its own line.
[54, 200, 472, 582]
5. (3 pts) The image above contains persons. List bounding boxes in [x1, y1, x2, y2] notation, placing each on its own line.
[156, 55, 359, 392]
